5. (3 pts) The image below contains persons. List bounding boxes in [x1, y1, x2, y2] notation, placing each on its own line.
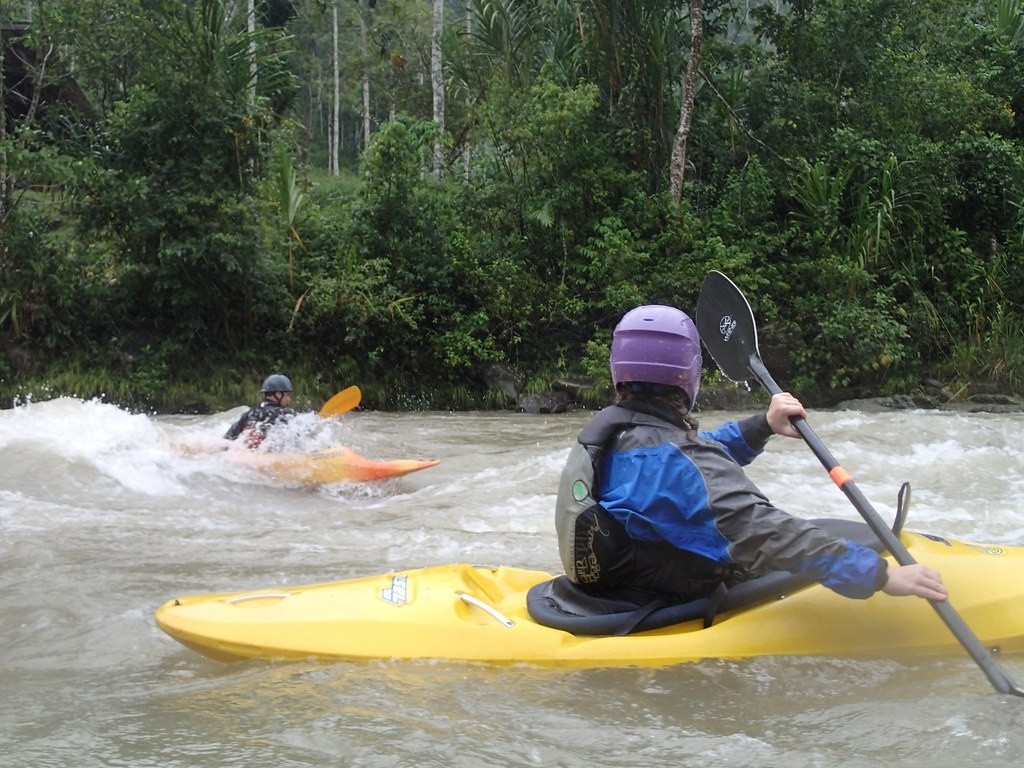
[223, 374, 301, 452]
[555, 303, 948, 607]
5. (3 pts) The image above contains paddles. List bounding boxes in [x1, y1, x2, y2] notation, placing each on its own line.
[694, 268, 1024, 698]
[314, 384, 363, 420]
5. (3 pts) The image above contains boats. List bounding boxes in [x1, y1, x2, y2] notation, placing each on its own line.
[211, 444, 438, 493]
[153, 521, 1024, 679]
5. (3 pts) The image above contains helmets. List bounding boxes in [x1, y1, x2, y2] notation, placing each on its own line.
[260, 373, 293, 391]
[609, 305, 703, 414]
